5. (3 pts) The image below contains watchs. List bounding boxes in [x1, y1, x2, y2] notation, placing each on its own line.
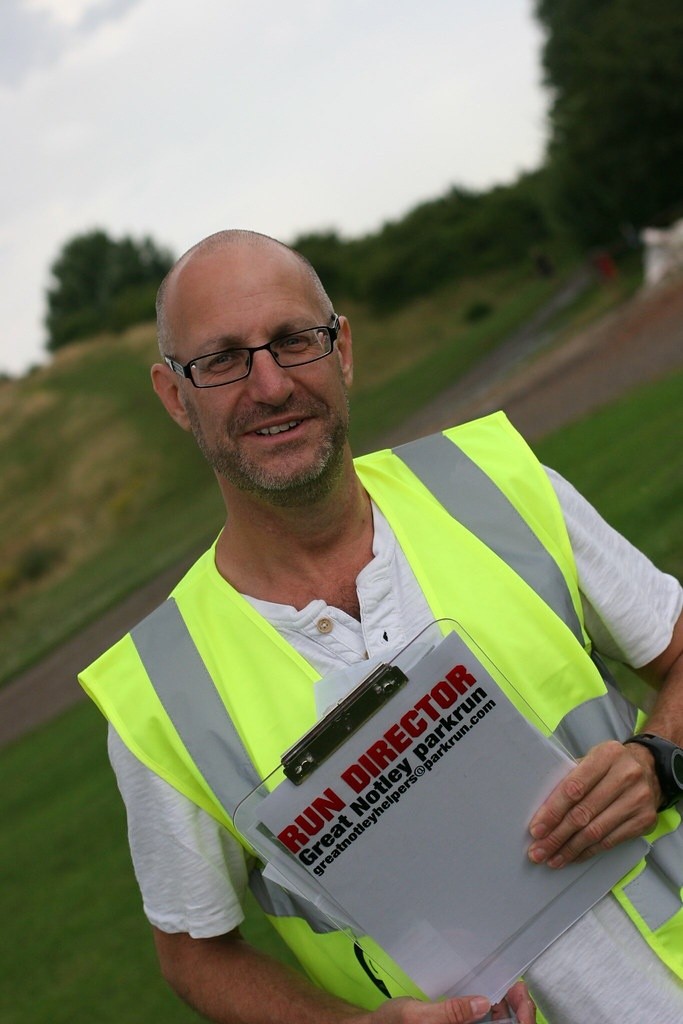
[623, 733, 683, 813]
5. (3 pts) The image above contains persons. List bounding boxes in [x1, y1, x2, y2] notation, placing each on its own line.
[76, 229, 683, 1024]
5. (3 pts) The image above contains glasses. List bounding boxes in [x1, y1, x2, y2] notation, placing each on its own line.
[165, 313, 341, 389]
